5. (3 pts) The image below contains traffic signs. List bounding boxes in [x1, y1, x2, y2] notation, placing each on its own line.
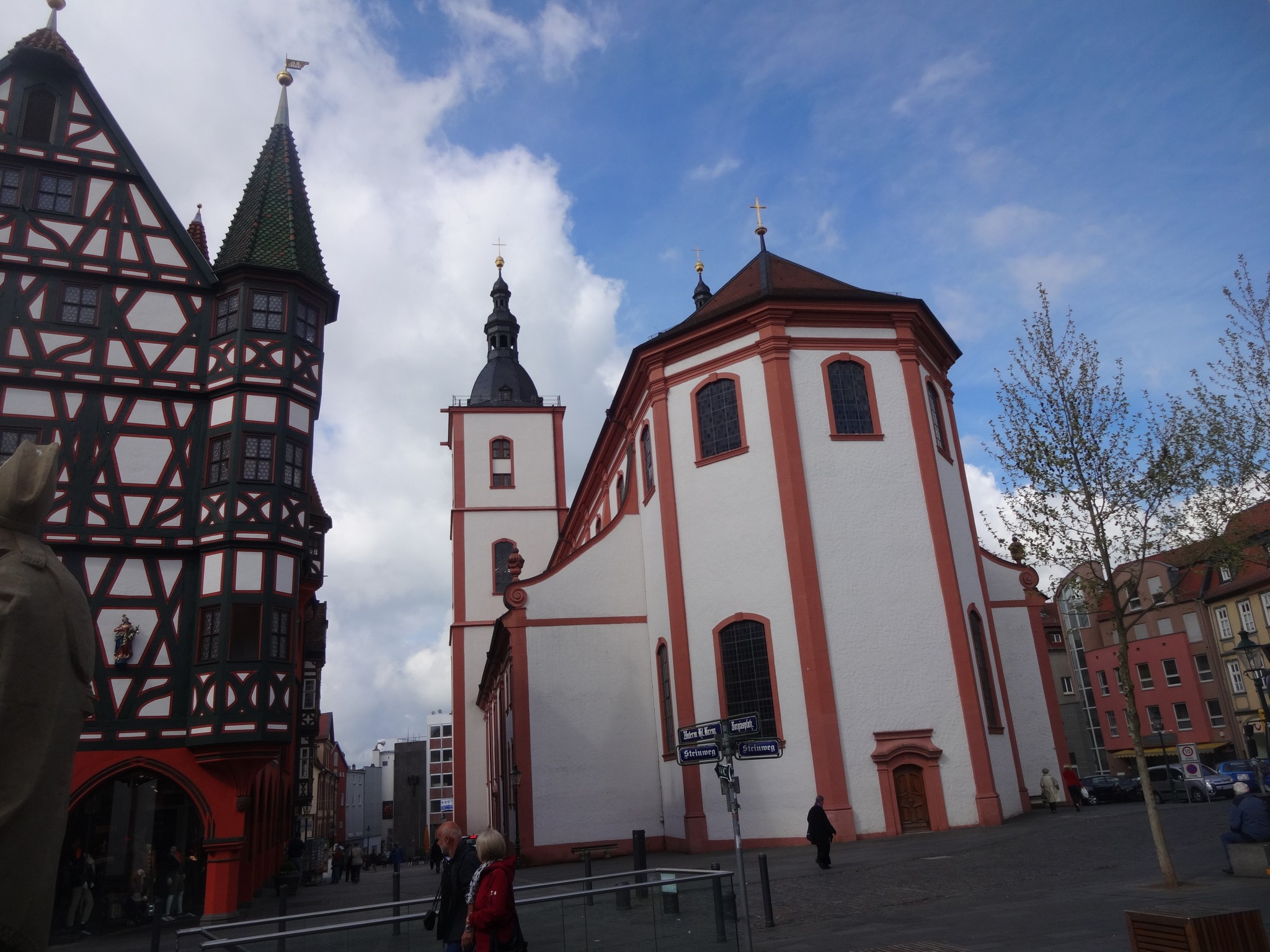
[713, 763, 732, 782]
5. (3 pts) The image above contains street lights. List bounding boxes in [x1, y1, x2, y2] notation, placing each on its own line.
[1150, 711, 1179, 804]
[1232, 623, 1270, 724]
[330, 825, 336, 852]
[366, 825, 371, 856]
[303, 804, 313, 842]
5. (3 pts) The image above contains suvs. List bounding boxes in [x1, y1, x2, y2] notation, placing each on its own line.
[1080, 759, 1270, 806]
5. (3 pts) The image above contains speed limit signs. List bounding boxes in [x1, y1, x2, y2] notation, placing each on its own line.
[1182, 746, 1193, 756]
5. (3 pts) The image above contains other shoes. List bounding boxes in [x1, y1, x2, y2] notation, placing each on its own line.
[436, 870, 440, 873]
[815, 858, 819, 864]
[1075, 803, 1080, 811]
[819, 863, 831, 869]
[1053, 810, 1057, 813]
[1222, 867, 1233, 874]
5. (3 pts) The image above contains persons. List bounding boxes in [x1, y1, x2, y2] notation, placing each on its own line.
[68, 822, 524, 952]
[1220, 782, 1270, 874]
[0, 435, 94, 952]
[807, 796, 838, 870]
[1040, 768, 1061, 813]
[113, 614, 136, 661]
[1062, 764, 1084, 812]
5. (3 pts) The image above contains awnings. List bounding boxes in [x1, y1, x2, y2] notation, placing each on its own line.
[1112, 742, 1231, 757]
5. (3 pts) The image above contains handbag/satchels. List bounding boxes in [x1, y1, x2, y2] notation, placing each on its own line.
[423, 910, 436, 931]
[1041, 792, 1047, 803]
[430, 860, 435, 870]
[1081, 787, 1089, 798]
[490, 913, 527, 952]
[347, 850, 353, 864]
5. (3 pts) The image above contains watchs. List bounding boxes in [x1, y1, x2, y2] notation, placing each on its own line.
[465, 925, 474, 932]
